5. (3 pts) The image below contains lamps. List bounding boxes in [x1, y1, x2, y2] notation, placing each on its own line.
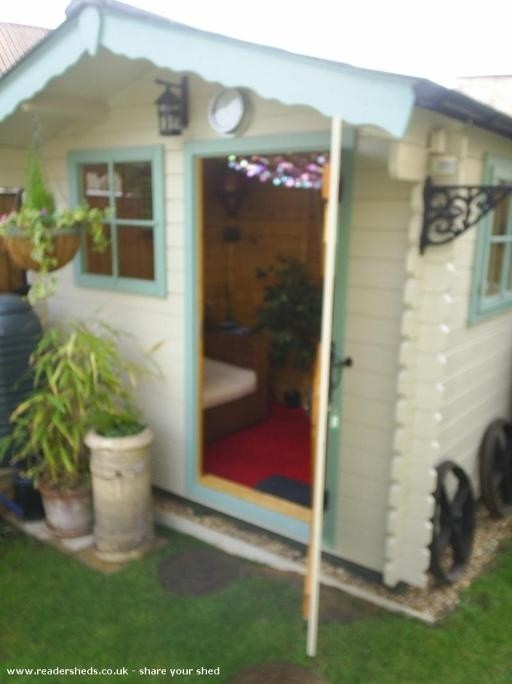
[153, 75, 188, 135]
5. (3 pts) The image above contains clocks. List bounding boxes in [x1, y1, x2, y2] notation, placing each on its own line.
[207, 85, 248, 139]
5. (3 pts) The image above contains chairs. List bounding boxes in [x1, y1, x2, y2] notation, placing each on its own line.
[204, 326, 273, 443]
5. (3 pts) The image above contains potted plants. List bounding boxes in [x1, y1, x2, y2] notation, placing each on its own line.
[0, 133, 113, 308]
[0, 315, 155, 563]
